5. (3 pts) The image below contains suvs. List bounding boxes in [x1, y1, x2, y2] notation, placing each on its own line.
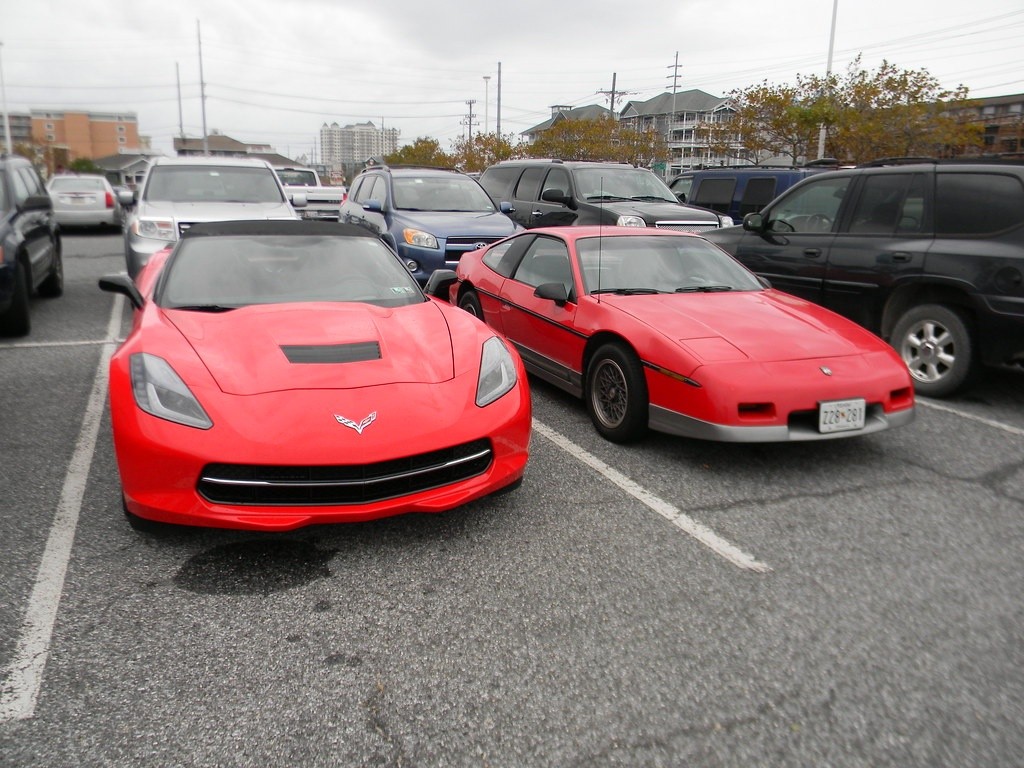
[338, 163, 527, 288]
[690, 156, 1023, 399]
[119, 153, 308, 281]
[476, 157, 734, 240]
[668, 157, 843, 235]
[0, 155, 63, 337]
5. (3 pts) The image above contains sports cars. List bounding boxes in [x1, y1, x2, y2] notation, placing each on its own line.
[450, 222, 915, 445]
[98, 218, 533, 534]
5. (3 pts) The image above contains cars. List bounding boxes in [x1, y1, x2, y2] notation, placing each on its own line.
[43, 173, 125, 235]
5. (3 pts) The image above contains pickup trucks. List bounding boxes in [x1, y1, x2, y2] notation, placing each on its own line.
[273, 164, 352, 224]
